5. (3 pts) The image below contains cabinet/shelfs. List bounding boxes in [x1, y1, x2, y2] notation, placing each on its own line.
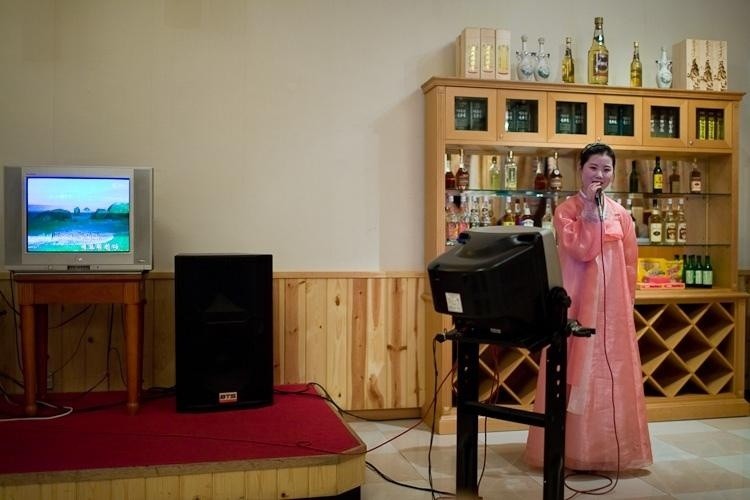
[421, 75, 750, 435]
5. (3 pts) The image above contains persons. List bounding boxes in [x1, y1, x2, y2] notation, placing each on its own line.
[521, 142, 653, 473]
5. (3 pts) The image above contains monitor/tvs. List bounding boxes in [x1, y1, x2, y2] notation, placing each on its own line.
[428, 228, 569, 340]
[4, 165, 153, 276]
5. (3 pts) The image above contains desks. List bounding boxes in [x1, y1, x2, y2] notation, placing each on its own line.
[14, 274, 146, 416]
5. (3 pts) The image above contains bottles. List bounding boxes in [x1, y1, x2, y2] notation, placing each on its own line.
[674, 254, 714, 289]
[446, 148, 563, 245]
[616, 155, 703, 245]
[514, 17, 674, 90]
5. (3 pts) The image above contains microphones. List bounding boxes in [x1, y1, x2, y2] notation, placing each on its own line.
[592, 189, 607, 223]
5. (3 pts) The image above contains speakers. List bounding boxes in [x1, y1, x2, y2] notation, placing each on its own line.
[174, 254, 273, 413]
